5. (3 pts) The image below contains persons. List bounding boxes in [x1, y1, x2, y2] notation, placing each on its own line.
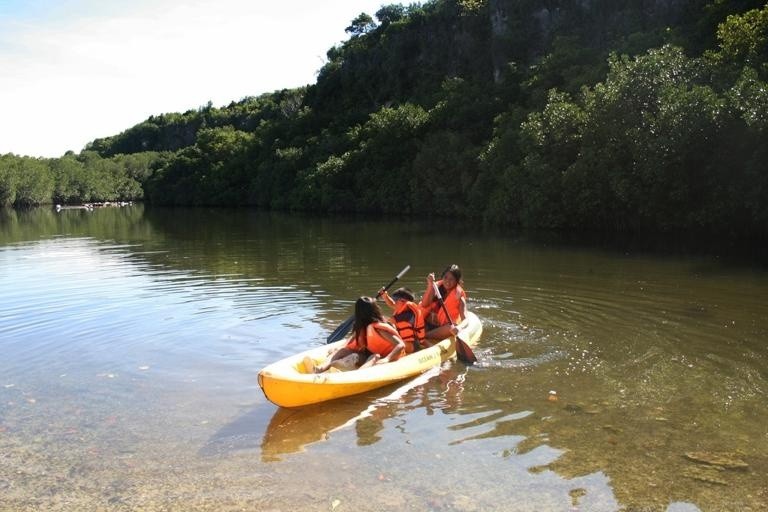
[419, 263, 469, 348]
[313, 295, 407, 373]
[376, 285, 426, 355]
[439, 370, 467, 416]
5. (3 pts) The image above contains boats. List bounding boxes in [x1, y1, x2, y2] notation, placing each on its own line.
[257, 310, 483, 408]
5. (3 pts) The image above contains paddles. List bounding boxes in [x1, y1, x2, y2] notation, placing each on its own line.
[432, 281, 477, 364]
[327, 267, 410, 343]
